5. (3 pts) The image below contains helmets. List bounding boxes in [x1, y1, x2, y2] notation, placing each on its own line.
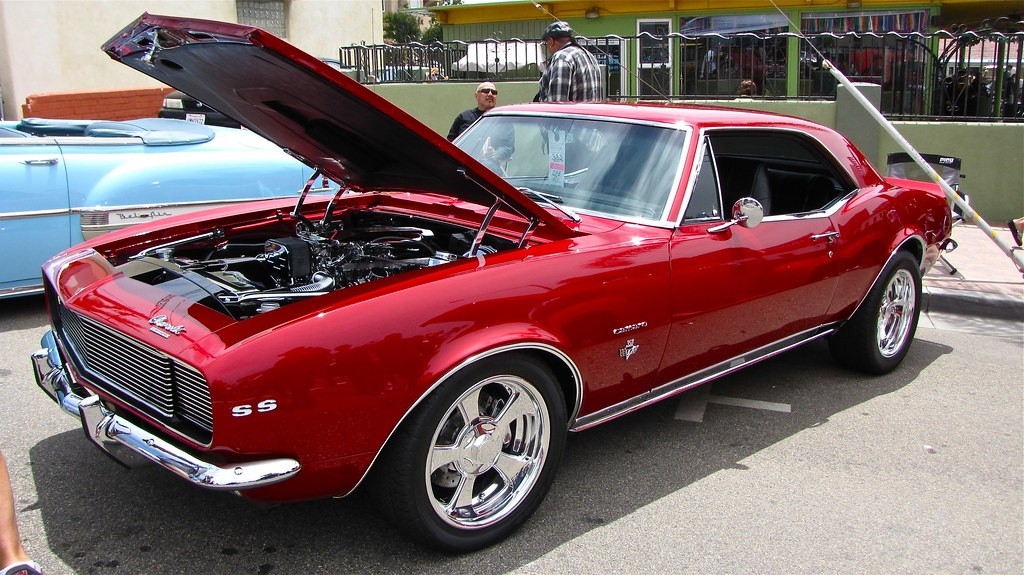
[542, 21, 573, 41]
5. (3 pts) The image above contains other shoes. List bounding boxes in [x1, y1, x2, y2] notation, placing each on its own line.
[0, 560, 42, 575]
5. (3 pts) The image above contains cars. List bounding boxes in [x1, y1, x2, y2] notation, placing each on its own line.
[33, 12, 953, 555]
[158, 58, 349, 129]
[0, 116, 348, 300]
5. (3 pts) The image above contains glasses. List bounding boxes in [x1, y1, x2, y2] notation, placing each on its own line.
[544, 36, 554, 45]
[477, 88, 497, 95]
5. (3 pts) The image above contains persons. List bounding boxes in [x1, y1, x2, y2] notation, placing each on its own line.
[426, 68, 448, 81]
[0, 448, 44, 575]
[972, 65, 1024, 117]
[739, 80, 757, 96]
[699, 47, 717, 92]
[446, 82, 516, 177]
[534, 22, 601, 187]
